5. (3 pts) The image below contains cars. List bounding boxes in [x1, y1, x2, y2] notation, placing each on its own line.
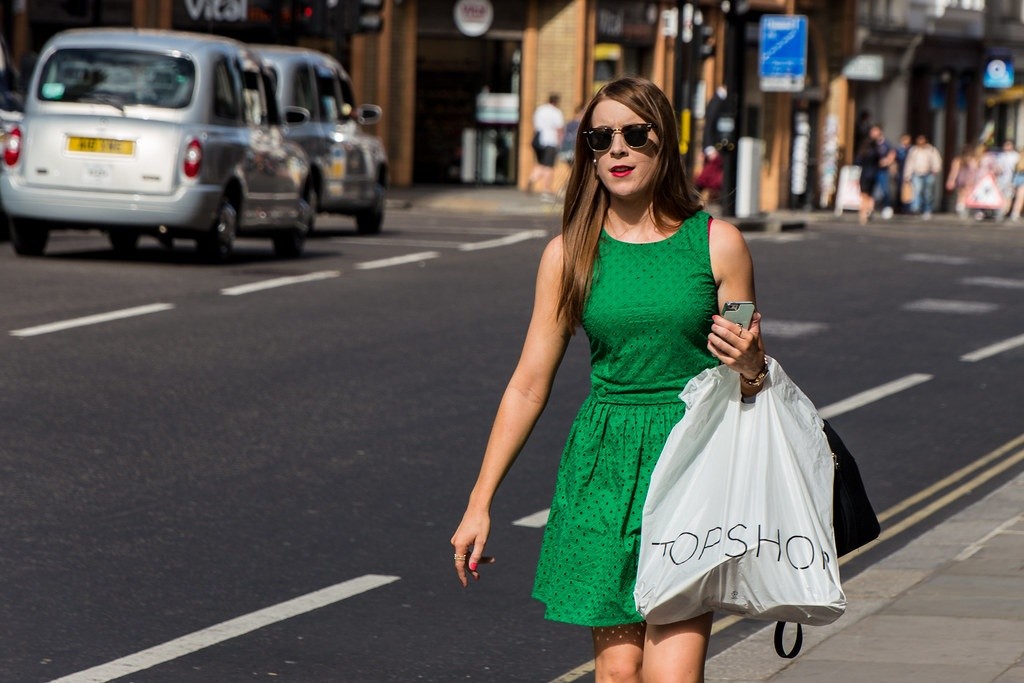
[0, 27, 324, 267]
[238, 43, 393, 235]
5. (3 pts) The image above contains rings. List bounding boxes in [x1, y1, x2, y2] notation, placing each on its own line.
[736, 323, 743, 336]
[454, 554, 466, 560]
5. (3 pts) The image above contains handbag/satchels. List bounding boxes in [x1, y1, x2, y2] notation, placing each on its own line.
[632, 352, 848, 625]
[773, 417, 882, 660]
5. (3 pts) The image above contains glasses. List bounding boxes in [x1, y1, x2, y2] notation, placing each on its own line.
[582, 122, 657, 152]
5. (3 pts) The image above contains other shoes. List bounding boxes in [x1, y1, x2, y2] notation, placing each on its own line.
[525, 181, 556, 204]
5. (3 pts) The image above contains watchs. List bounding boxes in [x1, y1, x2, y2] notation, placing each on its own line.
[740, 357, 769, 387]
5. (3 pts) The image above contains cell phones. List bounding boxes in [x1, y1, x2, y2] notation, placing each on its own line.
[720, 301, 755, 331]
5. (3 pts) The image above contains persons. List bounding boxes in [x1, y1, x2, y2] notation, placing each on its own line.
[947, 140, 1024, 222]
[856, 125, 942, 225]
[524, 94, 582, 202]
[703, 87, 734, 202]
[450, 77, 769, 683]
